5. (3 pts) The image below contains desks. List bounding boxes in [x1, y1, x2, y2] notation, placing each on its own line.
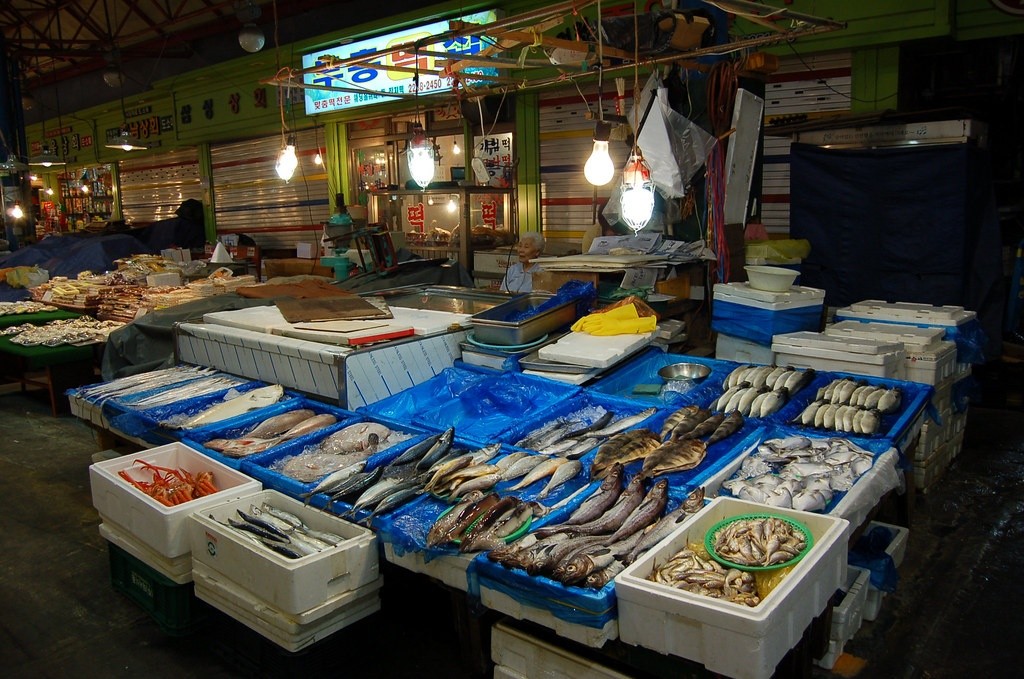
[0, 324, 104, 417]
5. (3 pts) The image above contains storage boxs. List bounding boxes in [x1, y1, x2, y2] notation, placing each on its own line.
[67, 280, 980, 678]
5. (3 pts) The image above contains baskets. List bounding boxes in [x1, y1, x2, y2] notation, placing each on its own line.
[437, 501, 533, 544]
[467, 330, 549, 351]
[702, 511, 812, 573]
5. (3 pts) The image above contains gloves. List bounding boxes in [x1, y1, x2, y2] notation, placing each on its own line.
[569, 303, 657, 336]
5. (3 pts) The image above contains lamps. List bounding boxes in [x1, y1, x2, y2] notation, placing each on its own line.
[102, 4, 148, 153]
[29, 1, 66, 167]
[237, 24, 265, 55]
[619, 2, 655, 235]
[407, 48, 434, 189]
[0, 152, 28, 174]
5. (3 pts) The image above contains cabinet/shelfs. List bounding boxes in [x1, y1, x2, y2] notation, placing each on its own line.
[63, 196, 111, 216]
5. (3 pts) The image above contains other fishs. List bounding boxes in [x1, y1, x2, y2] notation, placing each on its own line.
[74, 365, 904, 608]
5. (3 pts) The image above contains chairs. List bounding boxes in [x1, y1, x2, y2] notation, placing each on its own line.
[324, 222, 450, 286]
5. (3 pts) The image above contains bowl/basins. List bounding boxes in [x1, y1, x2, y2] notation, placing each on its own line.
[657, 362, 710, 384]
[743, 265, 801, 292]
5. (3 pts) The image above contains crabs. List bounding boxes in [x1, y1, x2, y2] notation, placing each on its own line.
[114, 457, 217, 513]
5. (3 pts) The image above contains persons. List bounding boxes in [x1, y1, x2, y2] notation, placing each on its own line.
[499, 232, 546, 292]
